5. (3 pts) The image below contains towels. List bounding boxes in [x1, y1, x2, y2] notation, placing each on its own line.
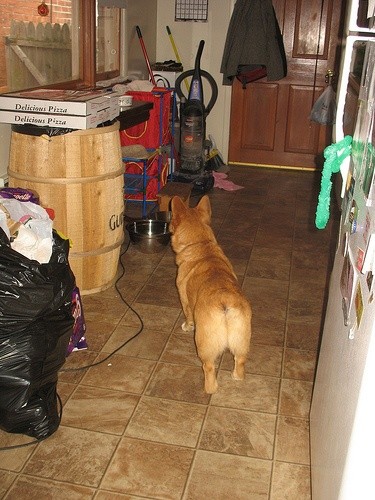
[210, 171, 244, 191]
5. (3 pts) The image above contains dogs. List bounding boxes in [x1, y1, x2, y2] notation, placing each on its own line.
[169, 194, 252, 394]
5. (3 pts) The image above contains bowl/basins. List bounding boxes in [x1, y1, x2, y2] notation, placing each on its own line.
[119, 96, 133, 106]
[125, 220, 173, 254]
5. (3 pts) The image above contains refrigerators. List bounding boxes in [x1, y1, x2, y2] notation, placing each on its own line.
[309, 0, 374, 500]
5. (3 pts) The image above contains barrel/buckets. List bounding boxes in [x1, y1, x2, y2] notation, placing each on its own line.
[8, 125, 124, 295]
